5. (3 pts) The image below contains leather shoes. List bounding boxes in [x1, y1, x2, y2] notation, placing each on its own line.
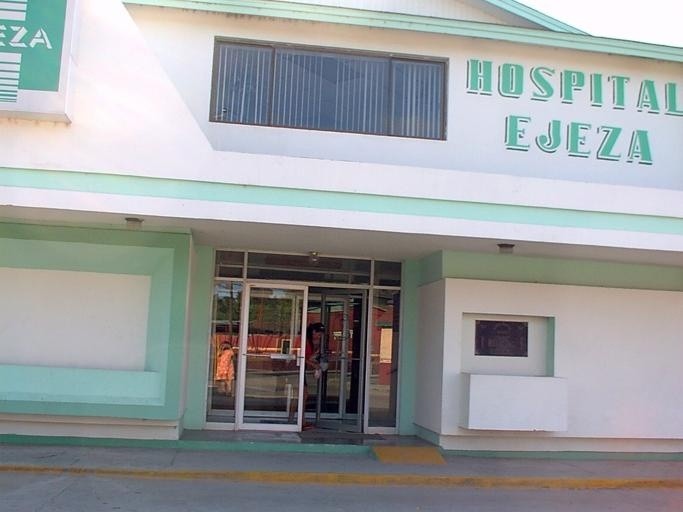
[302, 424, 314, 431]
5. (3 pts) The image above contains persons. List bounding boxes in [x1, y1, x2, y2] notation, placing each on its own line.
[286, 321, 324, 430]
[217, 341, 235, 396]
